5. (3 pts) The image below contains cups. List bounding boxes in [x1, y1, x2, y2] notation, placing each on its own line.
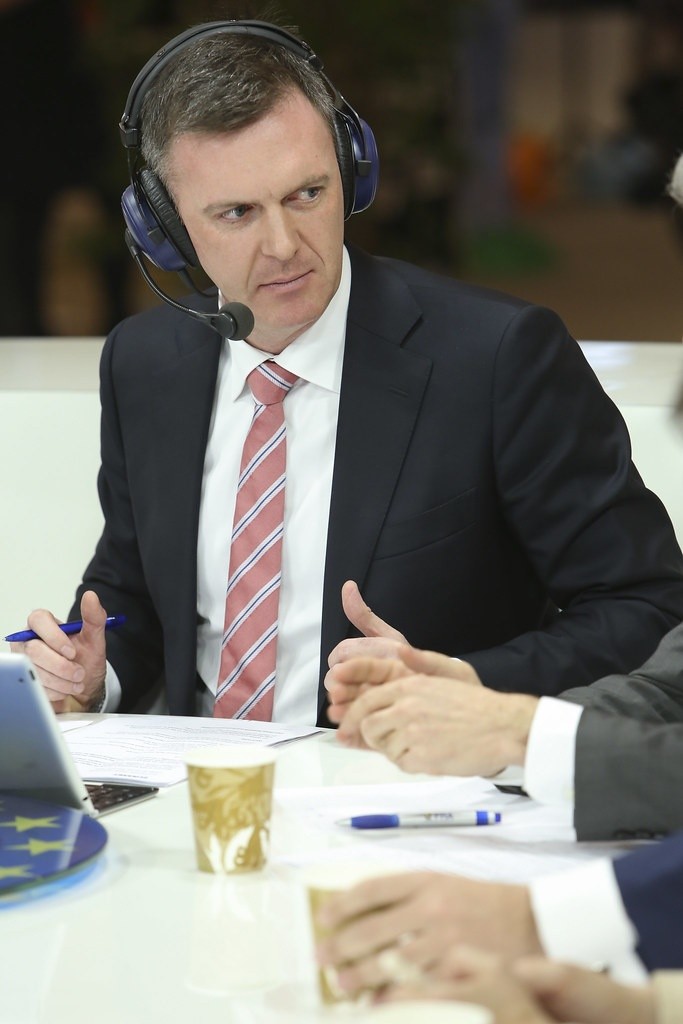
[308, 873, 378, 1007]
[188, 742, 277, 877]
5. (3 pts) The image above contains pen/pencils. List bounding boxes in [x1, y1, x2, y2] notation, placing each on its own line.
[335, 809, 503, 828]
[1, 613, 131, 642]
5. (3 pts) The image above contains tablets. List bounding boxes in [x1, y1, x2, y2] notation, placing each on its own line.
[0, 653, 98, 819]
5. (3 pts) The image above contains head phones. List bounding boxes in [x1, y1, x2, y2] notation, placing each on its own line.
[122, 20, 379, 276]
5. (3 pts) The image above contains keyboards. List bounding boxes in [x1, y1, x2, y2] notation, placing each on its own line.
[84, 784, 160, 815]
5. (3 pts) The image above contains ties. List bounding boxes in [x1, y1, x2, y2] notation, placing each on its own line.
[213, 359, 299, 723]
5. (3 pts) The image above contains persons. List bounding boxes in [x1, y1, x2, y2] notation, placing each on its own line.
[328, 622, 683, 843]
[9, 33, 682, 747]
[309, 833, 683, 1024]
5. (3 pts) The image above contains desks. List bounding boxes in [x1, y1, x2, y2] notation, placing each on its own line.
[0, 712, 683, 1023]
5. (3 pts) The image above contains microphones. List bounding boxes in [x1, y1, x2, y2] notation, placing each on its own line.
[133, 254, 255, 341]
